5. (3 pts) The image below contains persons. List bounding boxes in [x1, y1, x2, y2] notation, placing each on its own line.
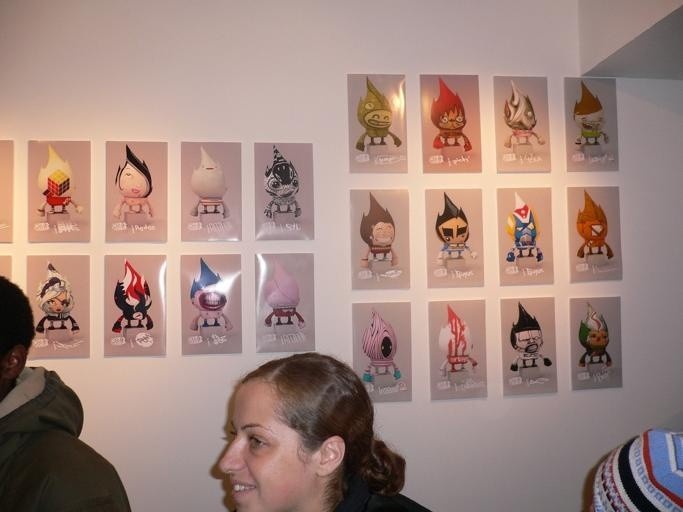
[0, 275, 133, 512]
[217, 351, 434, 512]
